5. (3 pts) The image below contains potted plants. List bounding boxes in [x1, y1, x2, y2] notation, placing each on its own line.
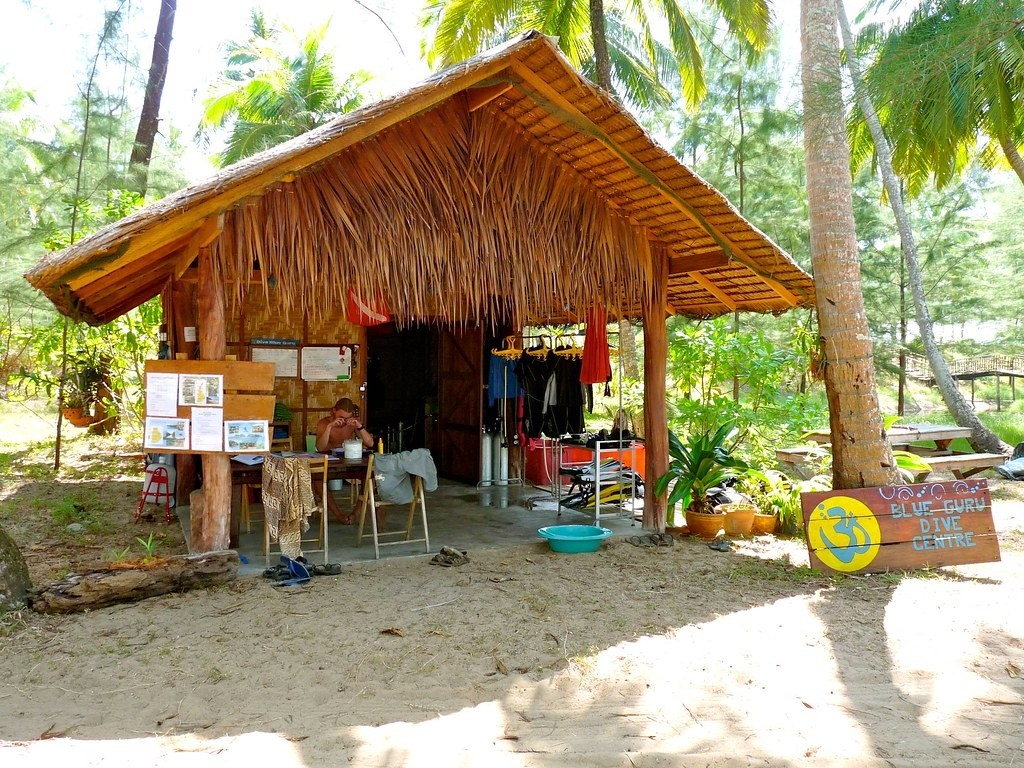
[653, 419, 794, 537]
[9, 344, 106, 428]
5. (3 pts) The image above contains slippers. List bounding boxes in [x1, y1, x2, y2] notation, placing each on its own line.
[625, 535, 644, 547]
[707, 535, 729, 552]
[647, 533, 664, 546]
[639, 535, 653, 547]
[661, 533, 674, 546]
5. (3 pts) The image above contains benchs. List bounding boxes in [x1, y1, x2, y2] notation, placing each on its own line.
[775, 443, 1009, 480]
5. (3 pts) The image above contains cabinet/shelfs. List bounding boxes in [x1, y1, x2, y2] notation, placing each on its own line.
[558, 435, 635, 526]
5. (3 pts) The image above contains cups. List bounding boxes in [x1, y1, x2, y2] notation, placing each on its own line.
[306, 432, 316, 454]
[344, 439, 363, 459]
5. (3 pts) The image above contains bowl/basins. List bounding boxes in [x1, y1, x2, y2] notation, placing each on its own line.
[537, 525, 612, 554]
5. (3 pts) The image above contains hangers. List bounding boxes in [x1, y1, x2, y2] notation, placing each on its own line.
[491, 333, 624, 361]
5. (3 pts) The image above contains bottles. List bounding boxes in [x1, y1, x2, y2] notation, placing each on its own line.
[240, 553, 257, 579]
[378, 438, 383, 455]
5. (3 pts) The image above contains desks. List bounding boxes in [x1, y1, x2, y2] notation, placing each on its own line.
[803, 426, 972, 482]
[241, 451, 386, 548]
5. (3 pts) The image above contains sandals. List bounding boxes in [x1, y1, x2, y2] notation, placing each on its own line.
[430, 547, 456, 565]
[440, 551, 470, 567]
[262, 556, 343, 579]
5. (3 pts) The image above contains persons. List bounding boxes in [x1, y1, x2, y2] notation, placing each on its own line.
[313, 398, 375, 525]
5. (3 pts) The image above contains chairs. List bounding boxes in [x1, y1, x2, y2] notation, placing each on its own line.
[234, 436, 430, 565]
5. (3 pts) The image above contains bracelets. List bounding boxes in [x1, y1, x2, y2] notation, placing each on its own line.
[357, 425, 365, 431]
